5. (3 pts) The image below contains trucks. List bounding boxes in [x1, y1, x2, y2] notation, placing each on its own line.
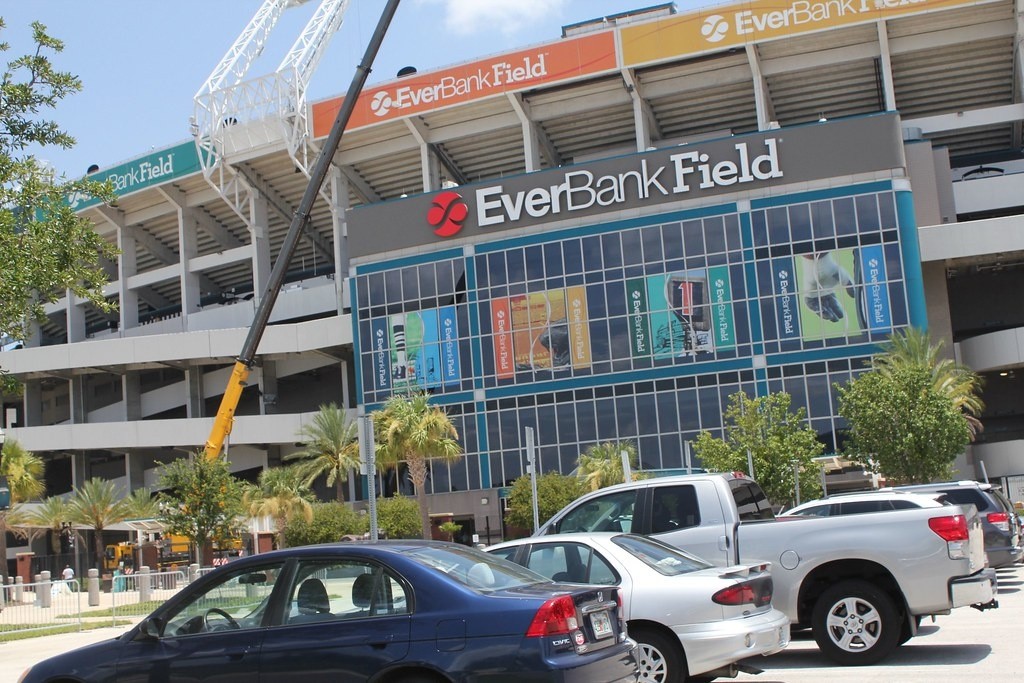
[104, 530, 242, 578]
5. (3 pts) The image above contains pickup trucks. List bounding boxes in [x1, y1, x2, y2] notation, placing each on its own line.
[530, 471, 1000, 666]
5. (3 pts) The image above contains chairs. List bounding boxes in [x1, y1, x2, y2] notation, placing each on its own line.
[651, 503, 676, 534]
[287, 578, 336, 625]
[551, 572, 570, 582]
[345, 573, 377, 619]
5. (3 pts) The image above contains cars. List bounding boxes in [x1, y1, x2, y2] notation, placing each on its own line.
[16, 539, 642, 683]
[340, 533, 790, 681]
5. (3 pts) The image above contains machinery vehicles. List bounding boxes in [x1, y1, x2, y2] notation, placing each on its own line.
[149, 0, 401, 561]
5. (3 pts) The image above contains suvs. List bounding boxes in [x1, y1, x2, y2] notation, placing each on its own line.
[877, 480, 1023, 569]
[778, 490, 954, 518]
